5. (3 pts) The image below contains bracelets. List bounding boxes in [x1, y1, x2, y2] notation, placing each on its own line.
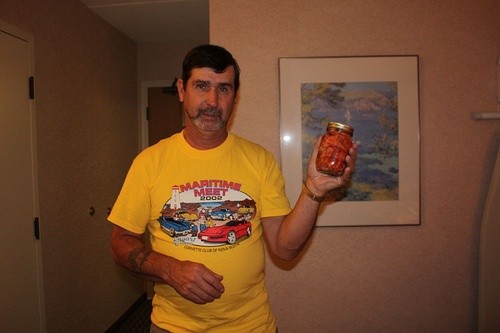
[301, 180, 325, 202]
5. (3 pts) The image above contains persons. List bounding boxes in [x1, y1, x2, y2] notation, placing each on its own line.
[107, 44, 358, 333]
